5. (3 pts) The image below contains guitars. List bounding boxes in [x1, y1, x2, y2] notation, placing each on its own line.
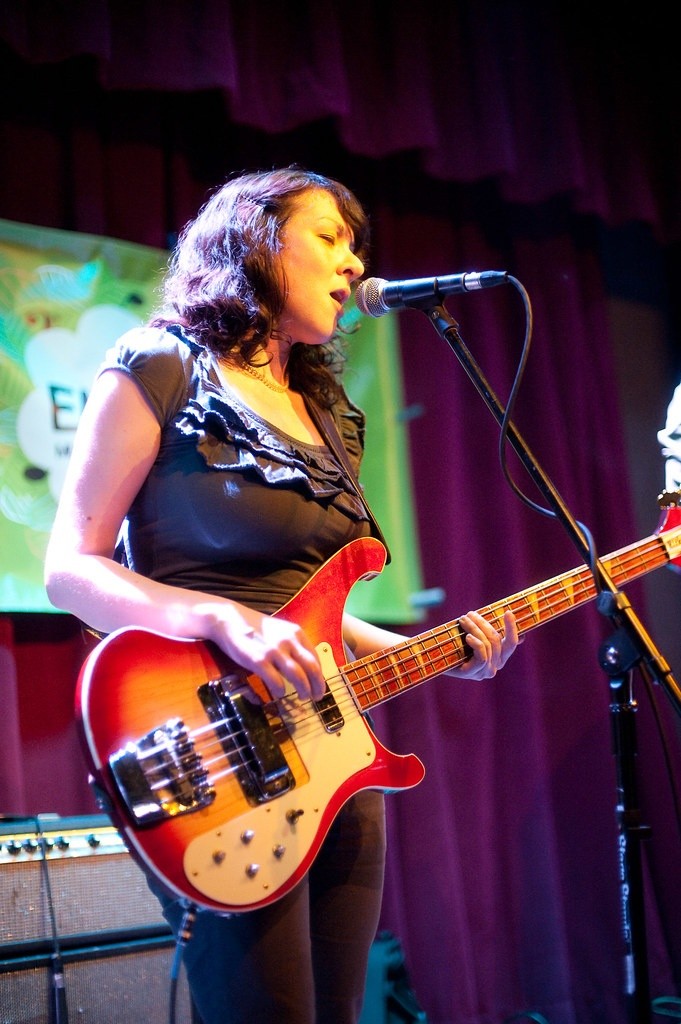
[70, 491, 680, 923]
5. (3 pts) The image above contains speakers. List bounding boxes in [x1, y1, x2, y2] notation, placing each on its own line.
[0, 933, 194, 1024]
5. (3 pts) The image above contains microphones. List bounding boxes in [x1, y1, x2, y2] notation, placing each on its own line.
[50, 952, 68, 1024]
[356, 269, 510, 317]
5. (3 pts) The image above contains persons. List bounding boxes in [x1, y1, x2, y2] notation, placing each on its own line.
[44, 171, 525, 1023]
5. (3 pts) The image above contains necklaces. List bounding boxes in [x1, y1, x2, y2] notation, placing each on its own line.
[228, 351, 291, 393]
